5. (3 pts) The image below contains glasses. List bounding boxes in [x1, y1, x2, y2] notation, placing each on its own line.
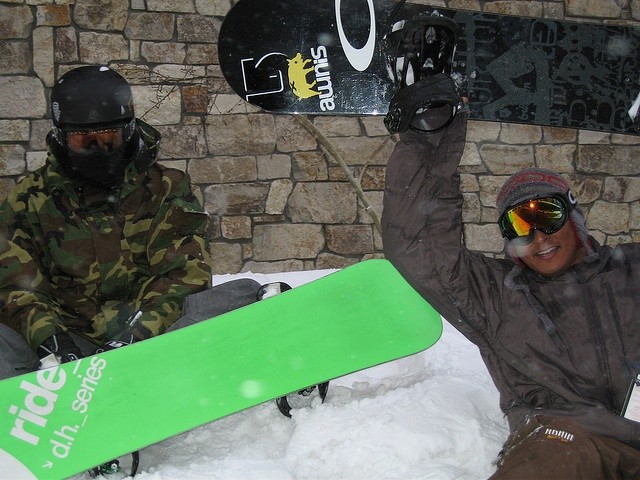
[57, 117, 136, 157]
[497, 188, 578, 246]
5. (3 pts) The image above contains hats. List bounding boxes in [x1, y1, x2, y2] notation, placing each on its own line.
[495, 168, 598, 270]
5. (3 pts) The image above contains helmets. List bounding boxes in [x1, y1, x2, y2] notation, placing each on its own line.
[50, 65, 135, 187]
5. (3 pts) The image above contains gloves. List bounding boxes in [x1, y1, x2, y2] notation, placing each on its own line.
[88, 332, 143, 356]
[36, 333, 87, 364]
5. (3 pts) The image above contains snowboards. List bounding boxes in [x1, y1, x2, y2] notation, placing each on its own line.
[0, 259, 443, 478]
[216, 0, 639, 139]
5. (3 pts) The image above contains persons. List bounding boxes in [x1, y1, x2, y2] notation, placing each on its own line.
[377, 4, 640, 480]
[0, 58, 337, 480]
